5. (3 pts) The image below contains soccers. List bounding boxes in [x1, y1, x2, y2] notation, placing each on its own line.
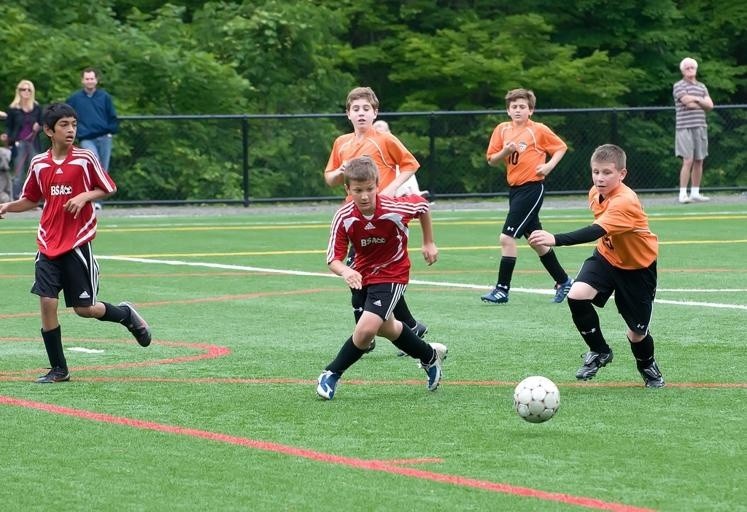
[513, 376, 561, 423]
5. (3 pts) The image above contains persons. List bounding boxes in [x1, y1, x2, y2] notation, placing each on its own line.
[315, 157, 448, 400]
[373, 120, 421, 198]
[65, 67, 118, 210]
[324, 86, 428, 357]
[672, 57, 714, 202]
[0, 102, 151, 383]
[0, 79, 42, 201]
[527, 143, 666, 390]
[479, 90, 574, 305]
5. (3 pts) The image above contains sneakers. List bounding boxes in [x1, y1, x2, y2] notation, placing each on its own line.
[396, 323, 427, 358]
[678, 192, 692, 204]
[635, 358, 667, 390]
[576, 346, 615, 379]
[118, 300, 153, 348]
[315, 370, 340, 402]
[420, 340, 447, 392]
[553, 278, 571, 304]
[481, 286, 510, 305]
[34, 365, 71, 385]
[689, 195, 710, 202]
